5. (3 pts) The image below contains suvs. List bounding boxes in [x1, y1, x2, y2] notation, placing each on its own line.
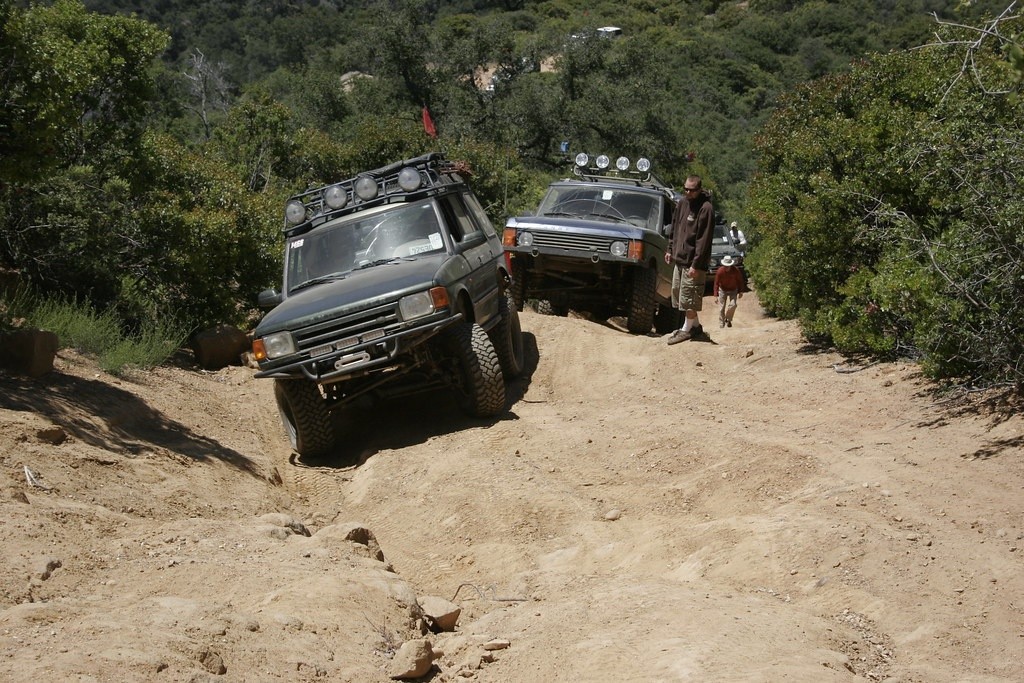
[251, 152, 524, 457]
[503, 151, 674, 334]
[707, 227, 746, 288]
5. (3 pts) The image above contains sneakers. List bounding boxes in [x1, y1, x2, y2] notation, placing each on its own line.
[667, 329, 691, 344]
[690, 324, 703, 336]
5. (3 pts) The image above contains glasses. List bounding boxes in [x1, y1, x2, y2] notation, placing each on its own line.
[683, 187, 699, 192]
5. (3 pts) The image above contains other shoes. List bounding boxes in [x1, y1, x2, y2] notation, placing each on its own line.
[719, 320, 725, 328]
[724, 318, 732, 327]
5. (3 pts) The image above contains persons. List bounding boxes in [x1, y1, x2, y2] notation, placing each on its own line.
[722, 222, 747, 257]
[713, 256, 744, 328]
[664, 175, 715, 345]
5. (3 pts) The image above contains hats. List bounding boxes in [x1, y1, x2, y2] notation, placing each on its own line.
[731, 222, 737, 227]
[721, 255, 734, 267]
